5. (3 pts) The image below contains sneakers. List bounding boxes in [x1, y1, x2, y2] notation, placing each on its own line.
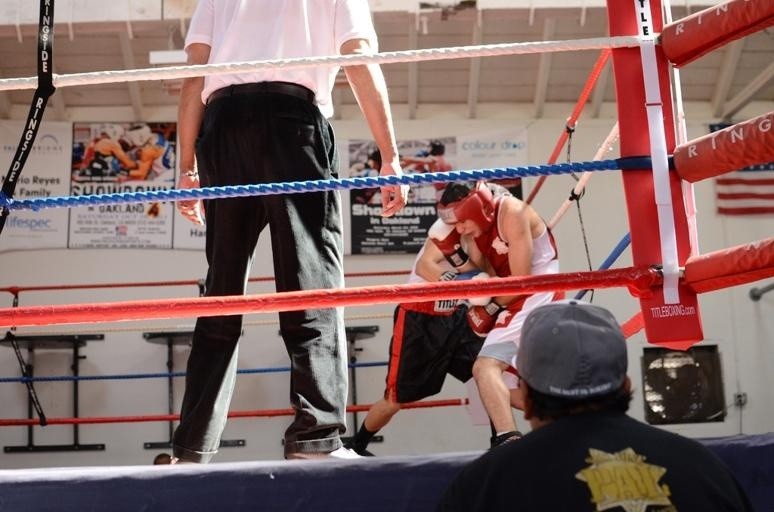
[491, 431, 522, 450]
[286, 447, 363, 459]
[343, 441, 375, 456]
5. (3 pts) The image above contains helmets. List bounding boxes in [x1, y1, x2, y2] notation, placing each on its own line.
[101, 125, 124, 140]
[368, 149, 382, 164]
[438, 182, 495, 234]
[429, 140, 444, 155]
[126, 124, 152, 147]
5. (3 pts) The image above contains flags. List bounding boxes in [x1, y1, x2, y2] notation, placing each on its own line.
[710, 122, 774, 215]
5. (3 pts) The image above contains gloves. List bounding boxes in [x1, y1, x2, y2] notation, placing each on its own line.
[451, 273, 492, 306]
[429, 218, 469, 269]
[467, 303, 507, 337]
[511, 300, 626, 397]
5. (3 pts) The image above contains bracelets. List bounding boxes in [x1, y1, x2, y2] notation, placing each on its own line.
[177, 171, 198, 177]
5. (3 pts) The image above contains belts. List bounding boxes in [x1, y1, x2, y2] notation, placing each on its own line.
[206, 82, 316, 105]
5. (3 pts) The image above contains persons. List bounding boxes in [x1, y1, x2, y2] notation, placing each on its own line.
[429, 179, 566, 450]
[166, 0, 409, 463]
[352, 146, 408, 204]
[436, 299, 754, 511]
[346, 182, 513, 456]
[400, 140, 453, 201]
[75, 123, 174, 183]
